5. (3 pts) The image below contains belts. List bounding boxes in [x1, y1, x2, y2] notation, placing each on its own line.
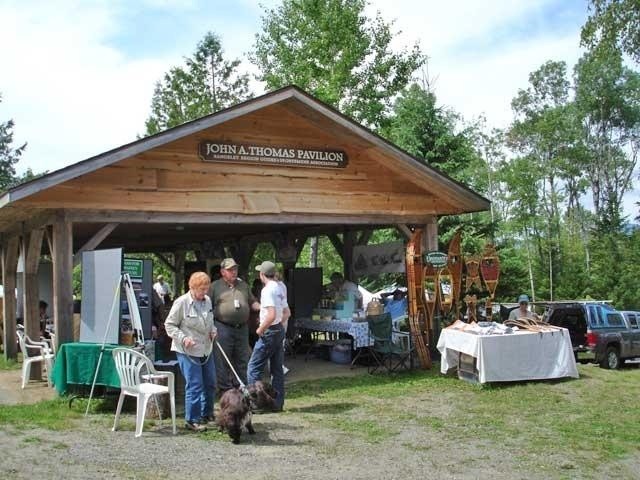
[213, 317, 246, 329]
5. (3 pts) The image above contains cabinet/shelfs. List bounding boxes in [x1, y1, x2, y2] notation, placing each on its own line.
[312, 288, 355, 319]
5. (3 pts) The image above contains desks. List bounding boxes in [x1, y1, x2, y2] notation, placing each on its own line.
[436, 322, 580, 384]
[293, 318, 375, 370]
[52, 341, 137, 397]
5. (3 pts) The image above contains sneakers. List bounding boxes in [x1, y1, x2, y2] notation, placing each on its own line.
[200, 415, 216, 422]
[185, 420, 207, 432]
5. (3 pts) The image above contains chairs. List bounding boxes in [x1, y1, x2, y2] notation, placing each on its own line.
[12, 322, 55, 389]
[109, 344, 179, 437]
[366, 312, 413, 376]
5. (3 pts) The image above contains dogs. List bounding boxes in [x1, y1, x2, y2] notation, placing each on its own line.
[215, 378, 280, 444]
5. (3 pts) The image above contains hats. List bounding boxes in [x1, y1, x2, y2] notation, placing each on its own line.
[220, 258, 240, 270]
[518, 295, 529, 303]
[255, 261, 276, 276]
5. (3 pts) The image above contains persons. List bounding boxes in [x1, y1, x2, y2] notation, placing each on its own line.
[149, 257, 293, 432]
[330, 272, 362, 309]
[39, 300, 52, 338]
[506, 293, 538, 322]
[72, 299, 82, 342]
[379, 289, 409, 320]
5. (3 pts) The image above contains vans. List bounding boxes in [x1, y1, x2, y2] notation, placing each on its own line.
[542, 301, 640, 369]
